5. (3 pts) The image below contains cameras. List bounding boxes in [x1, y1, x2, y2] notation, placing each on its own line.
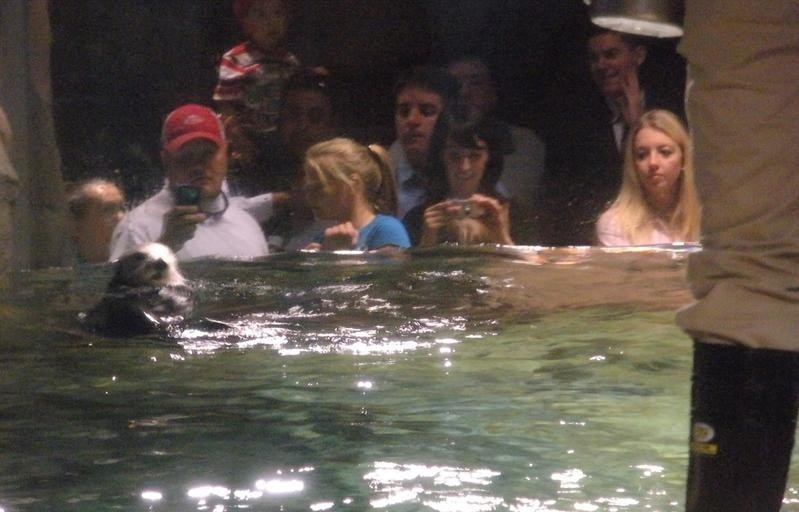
[445, 198, 481, 216]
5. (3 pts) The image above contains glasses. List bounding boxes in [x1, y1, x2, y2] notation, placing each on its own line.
[198, 190, 228, 219]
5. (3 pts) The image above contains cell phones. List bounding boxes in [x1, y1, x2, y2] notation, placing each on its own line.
[174, 183, 200, 205]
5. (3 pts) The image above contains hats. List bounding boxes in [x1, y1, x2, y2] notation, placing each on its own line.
[212, 40, 288, 100]
[162, 105, 227, 154]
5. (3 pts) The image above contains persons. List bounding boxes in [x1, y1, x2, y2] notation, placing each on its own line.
[669, 1, 799, 512]
[55, 0, 701, 266]
